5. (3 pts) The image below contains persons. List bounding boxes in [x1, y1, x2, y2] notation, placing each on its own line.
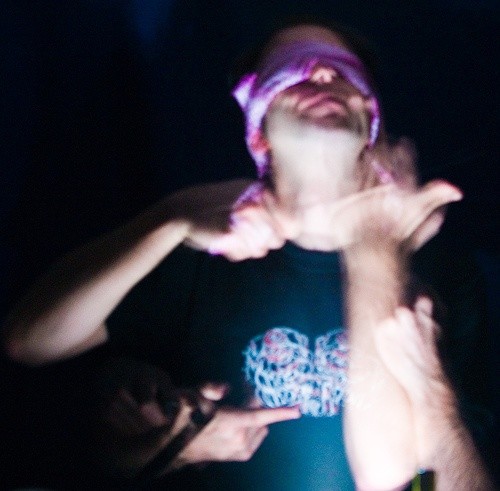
[56, 355, 301, 487]
[1, 23, 499, 491]
